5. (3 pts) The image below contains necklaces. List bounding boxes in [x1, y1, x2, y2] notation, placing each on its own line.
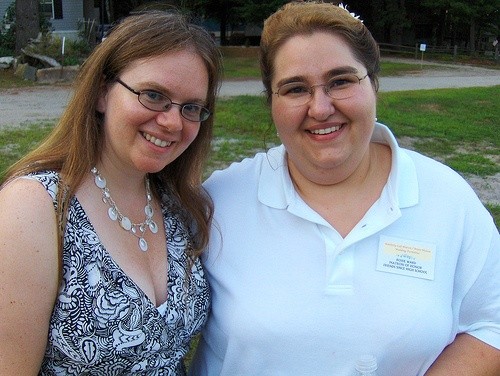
[91, 167, 158, 251]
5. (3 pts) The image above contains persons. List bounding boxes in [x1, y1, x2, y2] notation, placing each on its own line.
[0, 12, 224, 376]
[186, 1, 500, 376]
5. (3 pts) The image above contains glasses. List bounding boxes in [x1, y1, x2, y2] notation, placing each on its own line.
[115, 77, 213, 123]
[271, 74, 368, 106]
